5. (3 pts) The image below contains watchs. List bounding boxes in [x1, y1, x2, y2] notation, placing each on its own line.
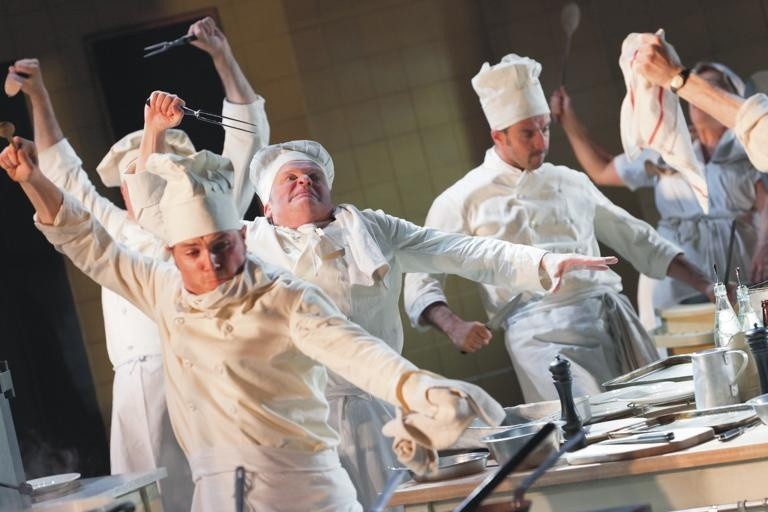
[669, 68, 690, 94]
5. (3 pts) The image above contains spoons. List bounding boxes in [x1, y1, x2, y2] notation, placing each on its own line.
[1, 121, 20, 152]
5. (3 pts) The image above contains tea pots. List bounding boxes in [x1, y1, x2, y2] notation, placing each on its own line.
[691, 346, 749, 410]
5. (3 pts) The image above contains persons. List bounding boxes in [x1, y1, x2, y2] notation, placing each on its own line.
[119, 88, 619, 512]
[0, 135, 473, 512]
[403, 52, 740, 405]
[546, 60, 768, 361]
[6, 13, 273, 512]
[634, 32, 768, 176]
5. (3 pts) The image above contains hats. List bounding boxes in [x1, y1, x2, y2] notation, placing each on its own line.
[96, 129, 243, 247]
[249, 139, 334, 207]
[470, 53, 551, 132]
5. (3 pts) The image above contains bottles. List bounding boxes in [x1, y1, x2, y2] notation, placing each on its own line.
[713, 261, 764, 349]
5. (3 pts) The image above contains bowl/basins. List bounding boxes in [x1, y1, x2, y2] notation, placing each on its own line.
[481, 419, 568, 472]
[745, 393, 768, 426]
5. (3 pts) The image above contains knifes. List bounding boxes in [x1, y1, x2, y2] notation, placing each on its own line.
[595, 431, 675, 444]
[4, 70, 30, 98]
[460, 291, 524, 354]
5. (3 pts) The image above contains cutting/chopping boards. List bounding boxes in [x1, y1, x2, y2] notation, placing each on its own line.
[566, 426, 714, 466]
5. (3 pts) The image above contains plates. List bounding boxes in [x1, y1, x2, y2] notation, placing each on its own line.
[26, 472, 82, 495]
[407, 451, 491, 484]
